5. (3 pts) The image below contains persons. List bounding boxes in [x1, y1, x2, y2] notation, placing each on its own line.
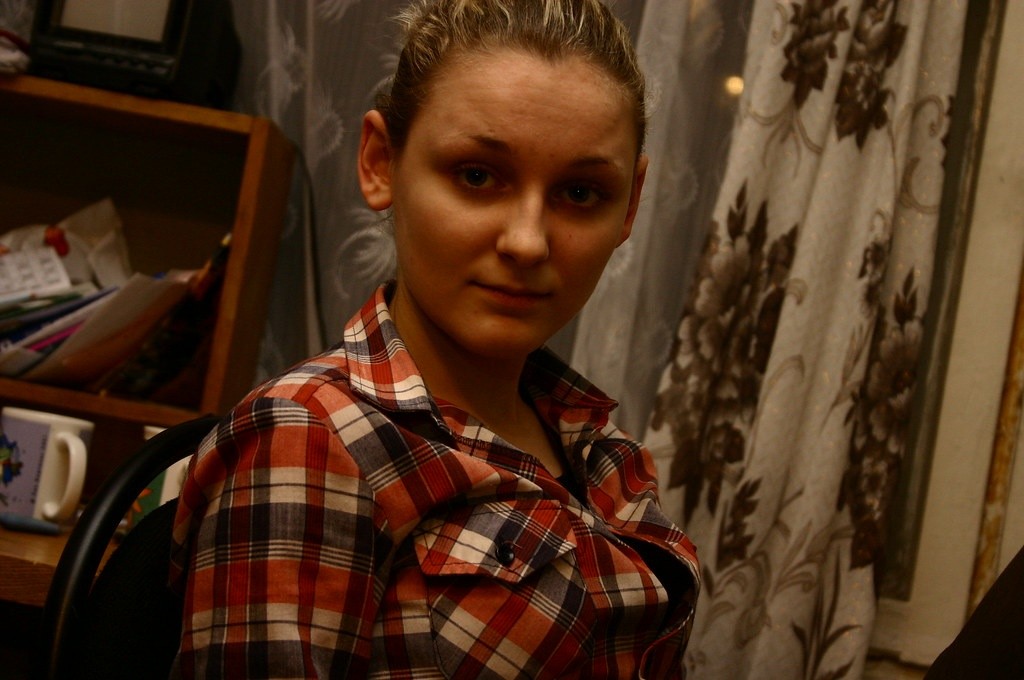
[168, 0, 702, 680]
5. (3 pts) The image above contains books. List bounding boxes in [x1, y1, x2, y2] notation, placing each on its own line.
[0, 226, 236, 399]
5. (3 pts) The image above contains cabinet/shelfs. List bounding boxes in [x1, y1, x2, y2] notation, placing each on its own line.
[0, 70, 295, 613]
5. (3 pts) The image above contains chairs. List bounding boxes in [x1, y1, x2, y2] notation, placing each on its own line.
[33, 416, 222, 679]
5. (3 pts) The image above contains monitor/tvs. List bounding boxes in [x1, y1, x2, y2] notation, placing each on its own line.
[26, 0, 244, 114]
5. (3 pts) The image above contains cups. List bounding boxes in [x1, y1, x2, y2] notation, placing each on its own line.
[127, 426, 193, 535]
[0, 407, 96, 534]
